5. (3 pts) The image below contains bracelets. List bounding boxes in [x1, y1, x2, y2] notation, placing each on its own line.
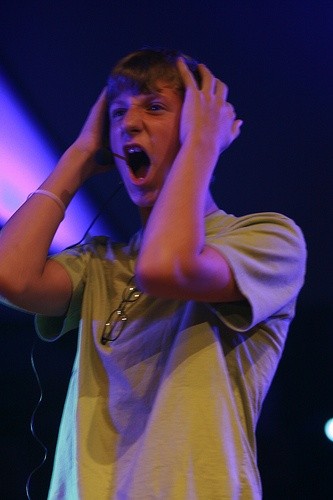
[27, 190, 67, 221]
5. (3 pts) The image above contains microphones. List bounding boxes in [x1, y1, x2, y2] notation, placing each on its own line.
[96, 147, 113, 165]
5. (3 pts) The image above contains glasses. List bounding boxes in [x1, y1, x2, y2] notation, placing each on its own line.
[100, 275, 145, 345]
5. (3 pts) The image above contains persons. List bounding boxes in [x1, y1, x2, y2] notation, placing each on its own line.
[0, 49, 306, 500]
